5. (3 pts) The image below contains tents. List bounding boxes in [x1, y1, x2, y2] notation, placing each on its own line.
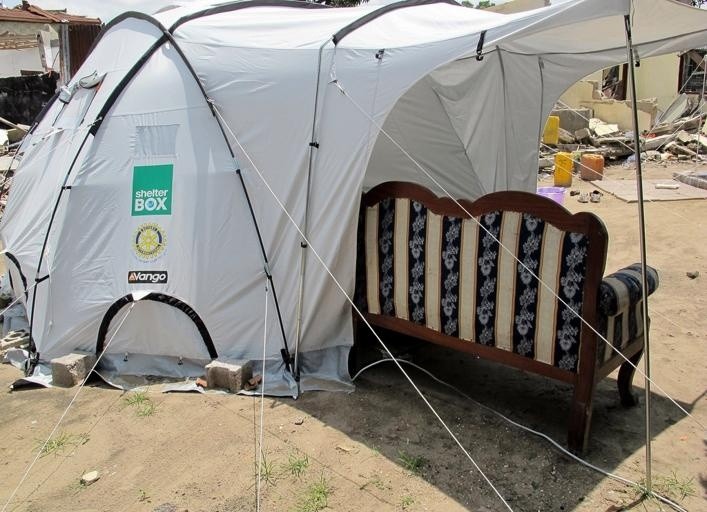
[1, 1, 706, 401]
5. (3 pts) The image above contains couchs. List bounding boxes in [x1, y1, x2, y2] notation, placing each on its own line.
[353, 180, 660, 455]
